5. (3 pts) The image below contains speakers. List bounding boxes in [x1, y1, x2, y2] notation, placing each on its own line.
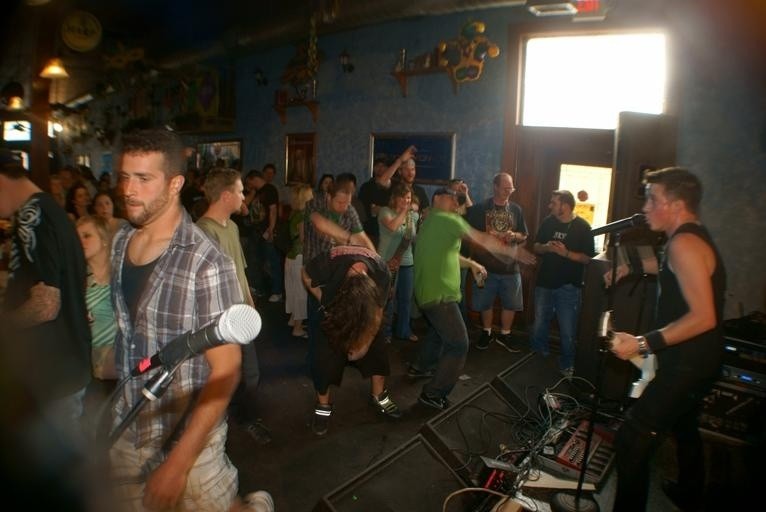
[493, 351, 585, 425]
[310, 433, 478, 512]
[416, 381, 542, 487]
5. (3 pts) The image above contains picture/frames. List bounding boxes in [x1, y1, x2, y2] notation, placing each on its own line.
[59, 9, 104, 54]
[368, 128, 458, 190]
[281, 130, 318, 189]
[195, 138, 243, 177]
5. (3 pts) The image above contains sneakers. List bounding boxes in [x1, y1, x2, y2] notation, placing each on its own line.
[406, 365, 436, 377]
[476, 329, 496, 349]
[496, 333, 521, 353]
[419, 391, 450, 410]
[366, 388, 401, 419]
[310, 402, 335, 436]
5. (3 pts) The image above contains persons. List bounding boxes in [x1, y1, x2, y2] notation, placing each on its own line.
[45, 144, 600, 440]
[0, 146, 92, 512]
[108, 118, 276, 512]
[602, 166, 727, 512]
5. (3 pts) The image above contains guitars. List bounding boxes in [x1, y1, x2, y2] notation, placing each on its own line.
[597, 311, 658, 400]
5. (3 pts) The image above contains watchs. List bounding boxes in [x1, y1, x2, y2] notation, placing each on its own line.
[636, 336, 649, 360]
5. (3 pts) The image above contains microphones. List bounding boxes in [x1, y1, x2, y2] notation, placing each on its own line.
[591, 213, 645, 237]
[131, 305, 262, 381]
